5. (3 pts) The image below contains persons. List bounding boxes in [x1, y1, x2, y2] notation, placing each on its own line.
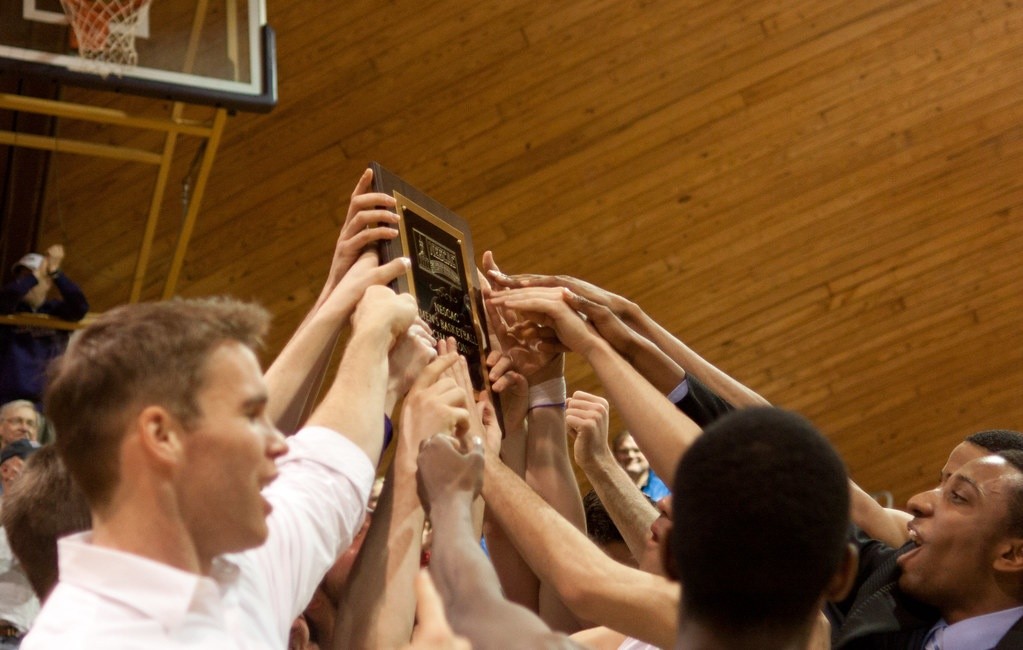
[0, 167, 1023, 650]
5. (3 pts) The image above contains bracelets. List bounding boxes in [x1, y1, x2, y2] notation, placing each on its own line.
[528, 376, 566, 411]
[47, 267, 60, 275]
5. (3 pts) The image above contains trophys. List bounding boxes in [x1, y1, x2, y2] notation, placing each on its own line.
[368, 160, 505, 439]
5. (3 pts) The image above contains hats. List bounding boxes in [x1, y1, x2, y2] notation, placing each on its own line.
[0, 439, 44, 463]
